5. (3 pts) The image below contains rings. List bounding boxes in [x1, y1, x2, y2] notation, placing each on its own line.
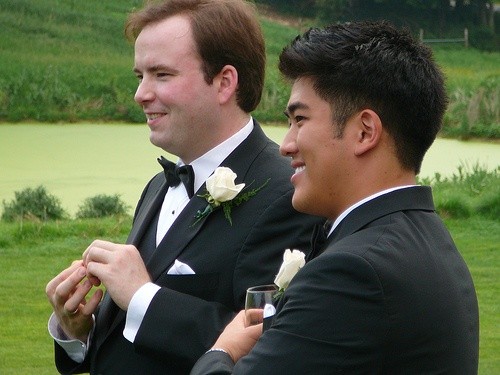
[64, 307, 80, 316]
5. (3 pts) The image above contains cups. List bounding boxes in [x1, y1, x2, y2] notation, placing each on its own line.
[244, 283, 279, 327]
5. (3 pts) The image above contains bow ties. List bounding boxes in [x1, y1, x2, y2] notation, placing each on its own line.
[305, 224, 327, 263]
[157, 155, 195, 199]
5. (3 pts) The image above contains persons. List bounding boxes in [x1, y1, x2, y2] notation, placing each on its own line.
[39, 0, 335, 375]
[191, 19, 480, 374]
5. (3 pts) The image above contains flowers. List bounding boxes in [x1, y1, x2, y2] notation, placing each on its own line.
[271, 249, 307, 305]
[188, 167, 271, 227]
